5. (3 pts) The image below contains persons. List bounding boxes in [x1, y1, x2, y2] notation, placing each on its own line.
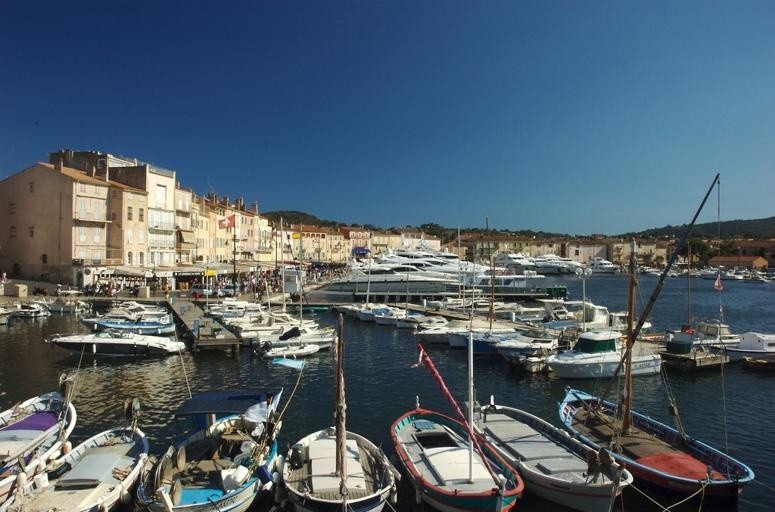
[56, 284, 63, 298]
[92, 264, 354, 302]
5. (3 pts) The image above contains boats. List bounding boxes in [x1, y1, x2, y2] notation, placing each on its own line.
[557, 172, 755, 510]
[1, 170, 337, 361]
[0, 344, 86, 498]
[0, 396, 150, 511]
[390, 333, 525, 511]
[463, 387, 634, 511]
[281, 313, 402, 512]
[134, 357, 307, 512]
[330, 242, 774, 381]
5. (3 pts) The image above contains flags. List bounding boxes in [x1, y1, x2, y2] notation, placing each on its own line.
[217, 215, 235, 229]
[272, 227, 277, 236]
[271, 356, 304, 369]
